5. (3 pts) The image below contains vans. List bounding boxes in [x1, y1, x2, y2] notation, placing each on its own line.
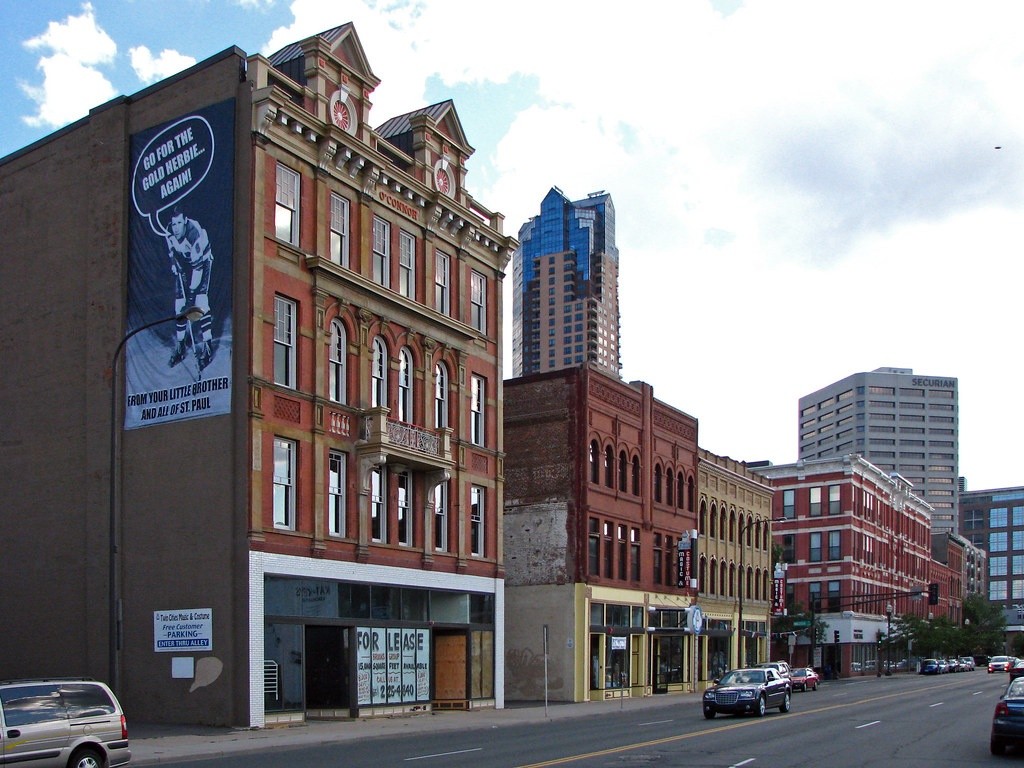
[0, 677, 132, 768]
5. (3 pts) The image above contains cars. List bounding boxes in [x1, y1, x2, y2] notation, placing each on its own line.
[752, 662, 791, 678]
[1010, 661, 1024, 685]
[1008, 657, 1019, 666]
[919, 659, 939, 675]
[937, 660, 949, 674]
[948, 660, 961, 672]
[785, 668, 821, 691]
[851, 662, 862, 672]
[864, 659, 906, 670]
[990, 677, 1024, 755]
[988, 656, 1009, 673]
[702, 669, 790, 718]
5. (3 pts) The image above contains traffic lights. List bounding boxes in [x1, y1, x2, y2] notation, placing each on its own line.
[928, 584, 938, 605]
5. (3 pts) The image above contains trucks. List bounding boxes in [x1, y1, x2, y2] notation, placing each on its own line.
[958, 657, 976, 672]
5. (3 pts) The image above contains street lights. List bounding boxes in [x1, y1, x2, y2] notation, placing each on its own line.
[964, 619, 969, 655]
[927, 613, 934, 658]
[885, 604, 893, 675]
[100, 305, 204, 754]
[736, 516, 786, 669]
